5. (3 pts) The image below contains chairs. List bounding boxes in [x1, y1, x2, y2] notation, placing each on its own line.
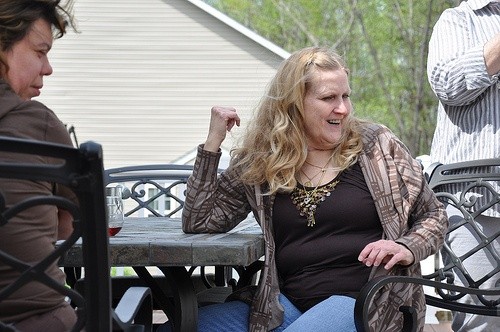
[354, 157, 496, 332]
[105, 165, 225, 218]
[0, 136, 111, 332]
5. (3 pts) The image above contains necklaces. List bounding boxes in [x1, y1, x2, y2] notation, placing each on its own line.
[288, 158, 333, 204]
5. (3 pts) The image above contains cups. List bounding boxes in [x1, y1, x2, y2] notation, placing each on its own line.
[105, 187, 124, 236]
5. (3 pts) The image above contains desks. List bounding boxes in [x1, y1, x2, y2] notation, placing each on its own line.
[56, 218, 263, 332]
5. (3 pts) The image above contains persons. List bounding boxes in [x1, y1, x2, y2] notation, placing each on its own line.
[0, 0, 86, 332]
[182, 46, 450, 332]
[425, 0, 500, 332]
